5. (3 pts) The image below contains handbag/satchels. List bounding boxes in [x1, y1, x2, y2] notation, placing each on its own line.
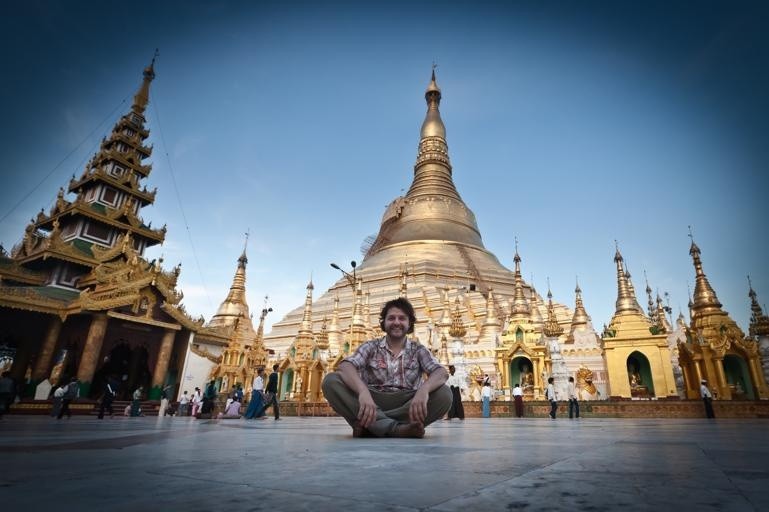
[195, 413, 212, 419]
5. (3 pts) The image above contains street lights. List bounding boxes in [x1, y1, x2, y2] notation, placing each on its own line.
[330, 260, 362, 355]
[458, 283, 476, 344]
[261, 307, 273, 317]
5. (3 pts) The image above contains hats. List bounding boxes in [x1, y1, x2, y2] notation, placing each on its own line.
[701, 379, 707, 383]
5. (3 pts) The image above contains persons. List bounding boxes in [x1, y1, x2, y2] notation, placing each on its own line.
[567, 376, 582, 418]
[544, 377, 558, 419]
[700, 379, 715, 418]
[444, 365, 465, 420]
[516, 358, 534, 398]
[512, 383, 524, 417]
[627, 357, 649, 398]
[483, 373, 489, 386]
[243, 366, 269, 419]
[481, 383, 490, 417]
[254, 364, 282, 420]
[1, 357, 242, 423]
[321, 296, 454, 440]
